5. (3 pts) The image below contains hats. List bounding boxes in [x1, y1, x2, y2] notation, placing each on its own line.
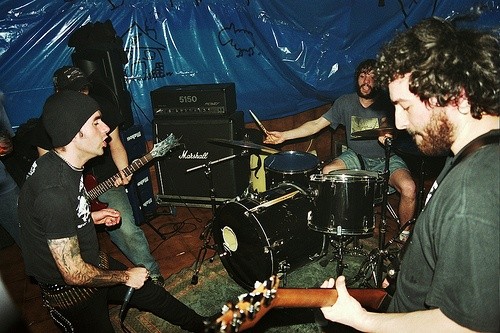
[41, 90, 101, 148]
[54, 65, 92, 93]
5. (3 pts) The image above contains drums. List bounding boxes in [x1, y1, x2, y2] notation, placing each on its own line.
[328, 168, 385, 204]
[213, 181, 326, 292]
[306, 173, 379, 237]
[263, 149, 320, 201]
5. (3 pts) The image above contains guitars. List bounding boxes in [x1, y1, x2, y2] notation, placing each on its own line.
[84, 131, 181, 212]
[208, 266, 398, 333]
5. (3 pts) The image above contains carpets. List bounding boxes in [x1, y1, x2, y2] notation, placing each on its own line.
[106, 235, 385, 333]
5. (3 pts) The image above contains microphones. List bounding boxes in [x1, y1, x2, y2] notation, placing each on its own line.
[118, 264, 145, 320]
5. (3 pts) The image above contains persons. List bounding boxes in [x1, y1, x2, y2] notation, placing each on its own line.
[320, 19, 500, 333]
[265, 59, 417, 239]
[0, 65, 227, 333]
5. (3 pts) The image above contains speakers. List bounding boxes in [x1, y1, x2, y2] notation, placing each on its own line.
[152, 111, 248, 208]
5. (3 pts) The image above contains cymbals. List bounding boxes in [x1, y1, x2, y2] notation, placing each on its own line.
[206, 138, 280, 154]
[350, 126, 410, 138]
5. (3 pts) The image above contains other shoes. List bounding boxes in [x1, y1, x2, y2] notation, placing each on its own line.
[399, 230, 410, 242]
[151, 275, 165, 287]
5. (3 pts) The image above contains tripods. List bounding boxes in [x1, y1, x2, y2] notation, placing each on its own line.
[182, 148, 251, 285]
[356, 135, 400, 290]
